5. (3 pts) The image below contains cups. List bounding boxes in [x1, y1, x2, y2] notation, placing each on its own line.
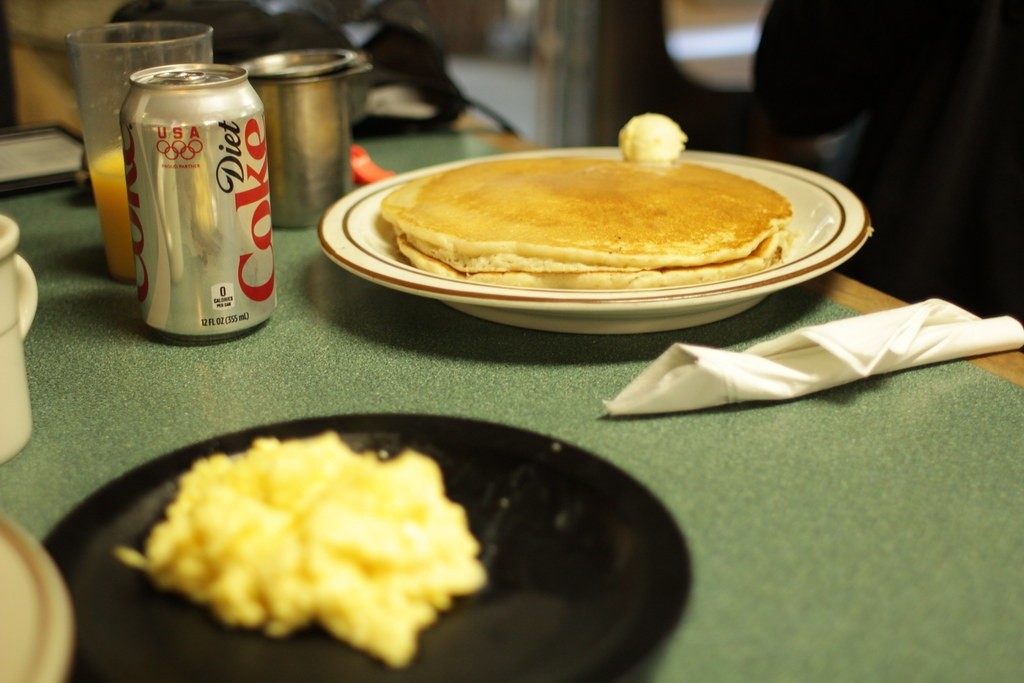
[0, 214, 40, 470]
[234, 49, 375, 232]
[64, 20, 216, 285]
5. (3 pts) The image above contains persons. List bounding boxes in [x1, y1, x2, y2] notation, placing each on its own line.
[751, 0, 1024, 320]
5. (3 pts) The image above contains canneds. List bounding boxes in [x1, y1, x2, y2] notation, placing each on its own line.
[118, 63, 276, 343]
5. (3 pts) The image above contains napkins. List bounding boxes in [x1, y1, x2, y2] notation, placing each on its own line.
[607, 297, 1024, 421]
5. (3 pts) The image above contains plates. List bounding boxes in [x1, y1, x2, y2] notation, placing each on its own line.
[0, 512, 74, 683]
[316, 145, 873, 335]
[44, 412, 687, 683]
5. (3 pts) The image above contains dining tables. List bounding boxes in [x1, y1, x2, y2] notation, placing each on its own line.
[1, 117, 1022, 683]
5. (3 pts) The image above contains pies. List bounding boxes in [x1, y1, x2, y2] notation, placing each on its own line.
[377, 155, 793, 291]
